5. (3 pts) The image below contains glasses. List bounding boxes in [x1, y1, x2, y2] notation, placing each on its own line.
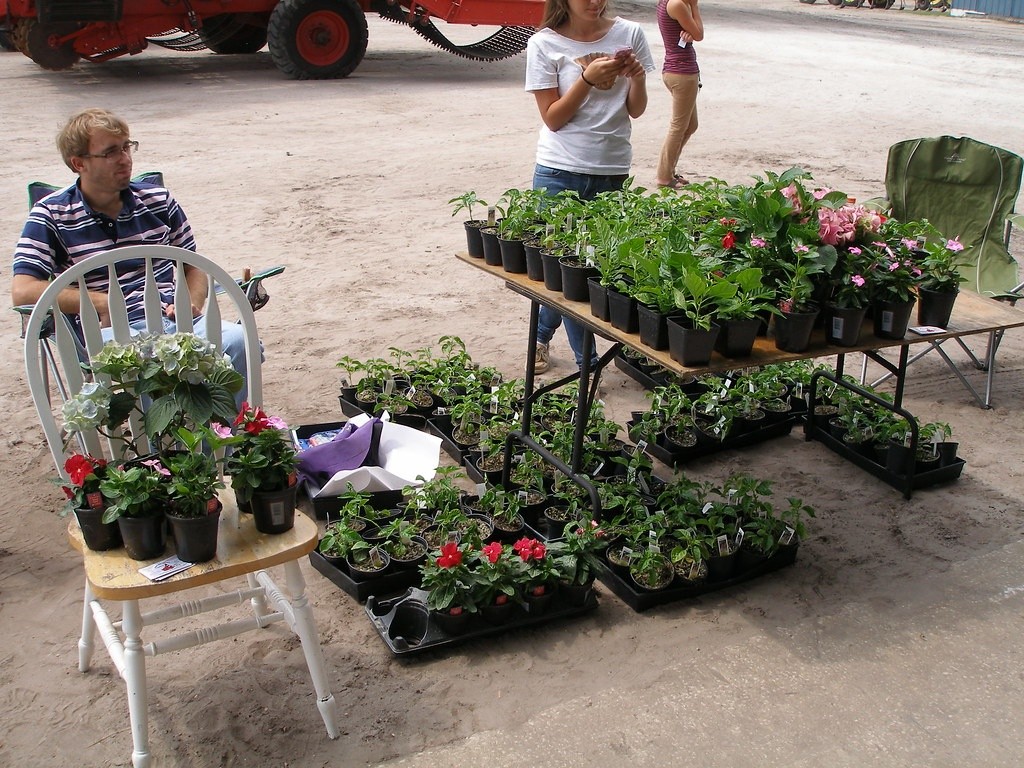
[75, 139, 139, 163]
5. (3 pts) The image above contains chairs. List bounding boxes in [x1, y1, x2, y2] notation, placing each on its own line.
[25, 244, 340, 767]
[10, 173, 286, 452]
[857, 136, 1023, 410]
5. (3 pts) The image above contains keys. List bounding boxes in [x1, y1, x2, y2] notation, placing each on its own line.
[698, 83, 702, 93]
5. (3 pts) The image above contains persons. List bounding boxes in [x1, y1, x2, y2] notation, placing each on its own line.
[525, 0, 655, 399]
[12, 107, 265, 475]
[656, 0, 704, 189]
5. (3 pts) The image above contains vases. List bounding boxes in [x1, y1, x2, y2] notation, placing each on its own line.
[919, 287, 960, 328]
[823, 297, 868, 347]
[227, 457, 254, 514]
[248, 477, 300, 535]
[164, 500, 223, 563]
[116, 513, 166, 561]
[435, 610, 471, 635]
[716, 314, 762, 360]
[560, 576, 595, 602]
[774, 301, 820, 351]
[122, 450, 191, 482]
[874, 295, 917, 341]
[481, 601, 512, 623]
[74, 507, 120, 551]
[521, 585, 555, 613]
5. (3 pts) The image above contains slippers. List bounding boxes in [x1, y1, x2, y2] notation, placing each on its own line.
[657, 179, 685, 190]
[673, 174, 689, 185]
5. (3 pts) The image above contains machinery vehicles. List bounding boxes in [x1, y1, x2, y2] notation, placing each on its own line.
[0, 0, 558, 80]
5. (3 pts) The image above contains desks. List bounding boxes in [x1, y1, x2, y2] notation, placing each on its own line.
[456, 252, 1023, 524]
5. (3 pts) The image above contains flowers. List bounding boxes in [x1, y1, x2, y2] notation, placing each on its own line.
[707, 169, 979, 328]
[58, 330, 303, 524]
[418, 516, 606, 614]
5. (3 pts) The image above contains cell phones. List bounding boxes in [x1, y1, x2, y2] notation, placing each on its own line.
[612, 47, 633, 67]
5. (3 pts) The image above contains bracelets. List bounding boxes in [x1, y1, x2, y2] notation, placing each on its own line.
[582, 71, 595, 86]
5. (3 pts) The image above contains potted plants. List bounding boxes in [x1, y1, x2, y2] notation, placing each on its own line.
[448, 175, 738, 331]
[317, 335, 958, 591]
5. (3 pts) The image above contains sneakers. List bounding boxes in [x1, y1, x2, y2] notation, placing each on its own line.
[586, 371, 603, 404]
[524, 341, 550, 375]
